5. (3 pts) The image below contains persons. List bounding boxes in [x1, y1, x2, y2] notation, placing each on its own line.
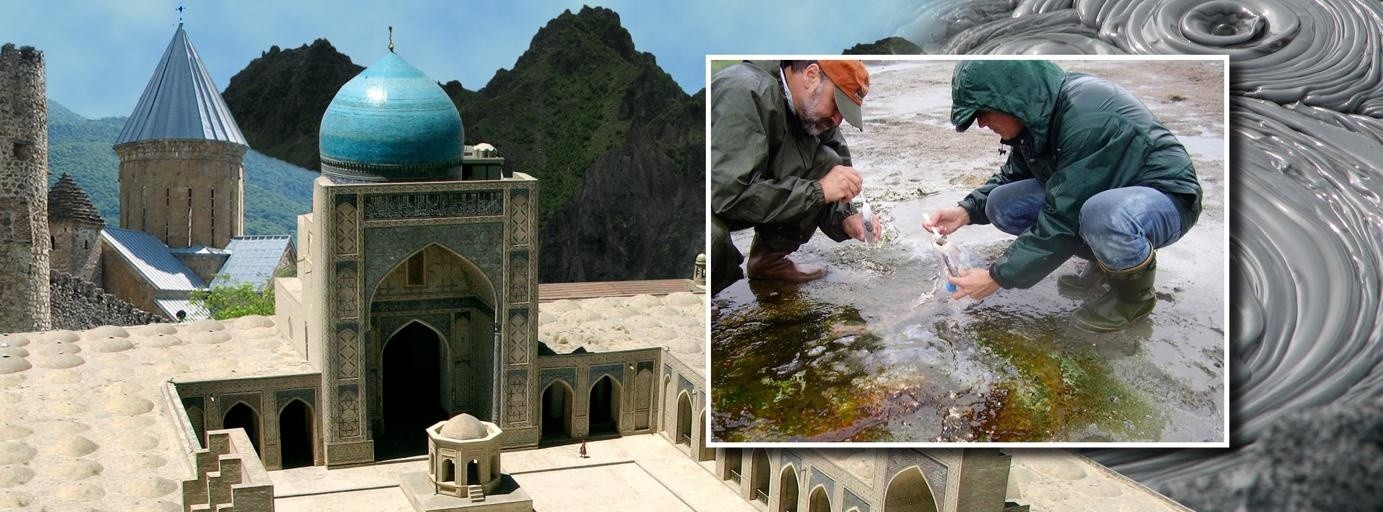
[922, 62, 1205, 333]
[712, 58, 883, 298]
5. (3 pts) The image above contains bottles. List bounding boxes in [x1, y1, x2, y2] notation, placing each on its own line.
[932, 235, 963, 290]
[861, 215, 877, 254]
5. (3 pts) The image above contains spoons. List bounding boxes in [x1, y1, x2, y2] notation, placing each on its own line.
[860, 190, 873, 222]
[921, 210, 948, 244]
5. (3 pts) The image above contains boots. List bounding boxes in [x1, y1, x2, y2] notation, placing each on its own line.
[1059, 238, 1157, 335]
[747, 236, 826, 290]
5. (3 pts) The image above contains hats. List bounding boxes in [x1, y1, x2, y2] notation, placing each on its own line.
[818, 60, 869, 132]
